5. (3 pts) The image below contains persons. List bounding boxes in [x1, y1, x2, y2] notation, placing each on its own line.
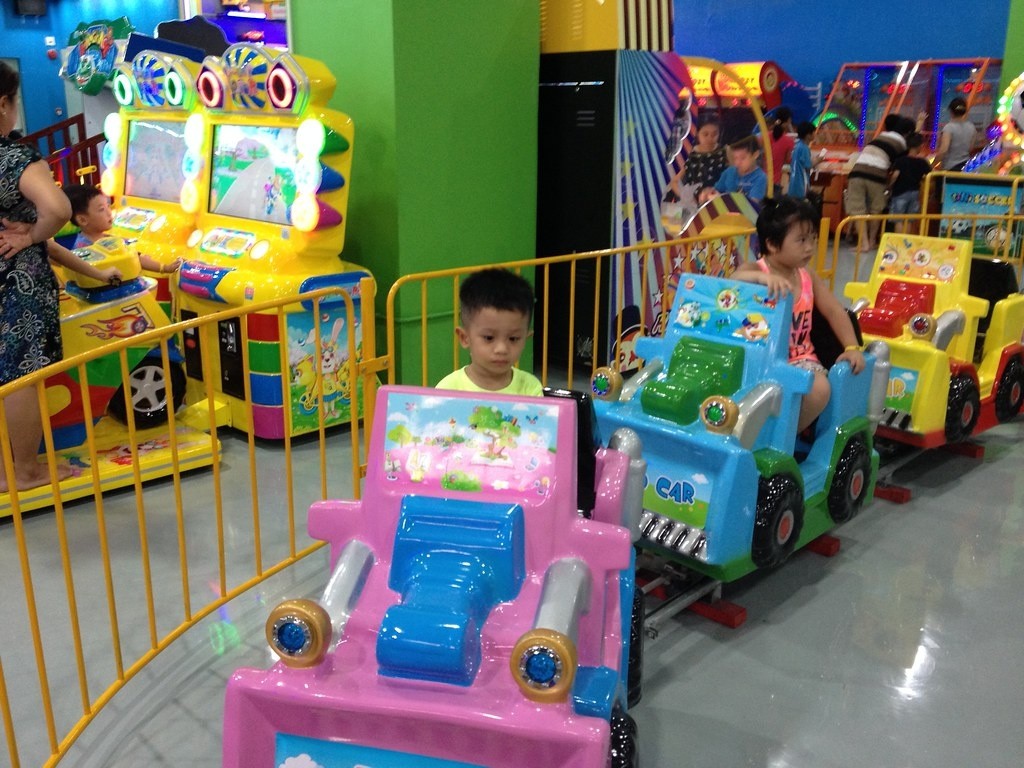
[435, 266, 543, 399]
[0, 59, 72, 493]
[48, 184, 186, 286]
[845, 97, 977, 253]
[729, 197, 865, 436]
[671, 107, 824, 260]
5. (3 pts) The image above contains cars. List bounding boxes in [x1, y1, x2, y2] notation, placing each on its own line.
[843, 232, 1024, 448]
[590, 271, 890, 587]
[219, 383, 648, 768]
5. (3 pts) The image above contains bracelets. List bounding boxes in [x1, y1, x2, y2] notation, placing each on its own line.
[160, 262, 165, 274]
[845, 346, 865, 352]
